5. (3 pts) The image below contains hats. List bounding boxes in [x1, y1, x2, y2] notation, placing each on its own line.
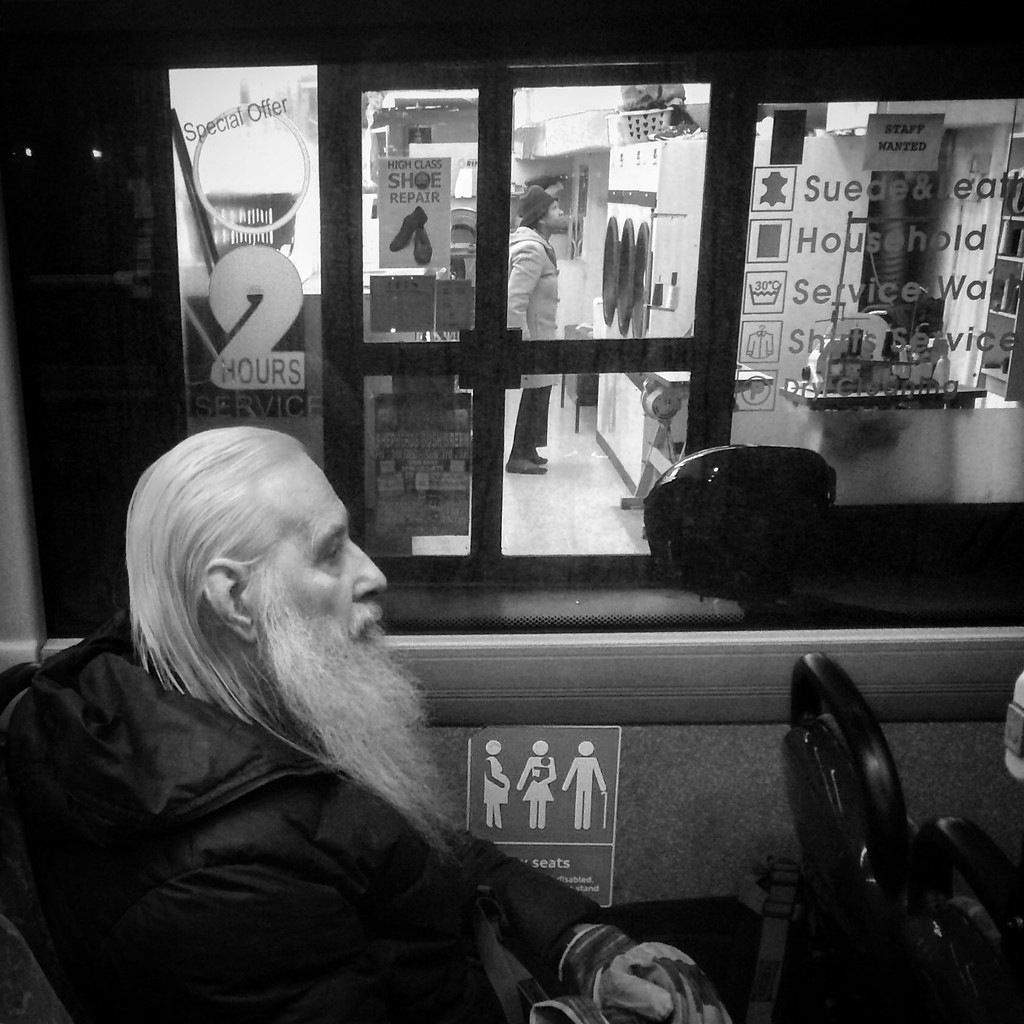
[519, 185, 558, 227]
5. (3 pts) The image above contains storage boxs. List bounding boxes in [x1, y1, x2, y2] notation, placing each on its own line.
[605, 108, 674, 144]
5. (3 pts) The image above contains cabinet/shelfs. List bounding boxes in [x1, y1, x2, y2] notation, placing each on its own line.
[973, 99, 1024, 404]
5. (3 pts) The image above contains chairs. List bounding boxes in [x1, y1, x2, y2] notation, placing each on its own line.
[902, 816, 1024, 1024]
[781, 653, 909, 1024]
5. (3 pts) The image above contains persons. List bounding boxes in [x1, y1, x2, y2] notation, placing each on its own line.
[17, 426, 736, 1023]
[506, 186, 563, 475]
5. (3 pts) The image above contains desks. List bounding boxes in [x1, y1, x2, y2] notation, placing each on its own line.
[780, 382, 986, 411]
[620, 362, 774, 539]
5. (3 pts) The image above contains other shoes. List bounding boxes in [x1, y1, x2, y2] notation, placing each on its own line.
[505, 455, 548, 474]
[390, 206, 427, 251]
[525, 446, 548, 464]
[413, 225, 432, 263]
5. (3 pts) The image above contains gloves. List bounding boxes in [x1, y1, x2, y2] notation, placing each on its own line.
[530, 995, 662, 1024]
[558, 924, 732, 1024]
